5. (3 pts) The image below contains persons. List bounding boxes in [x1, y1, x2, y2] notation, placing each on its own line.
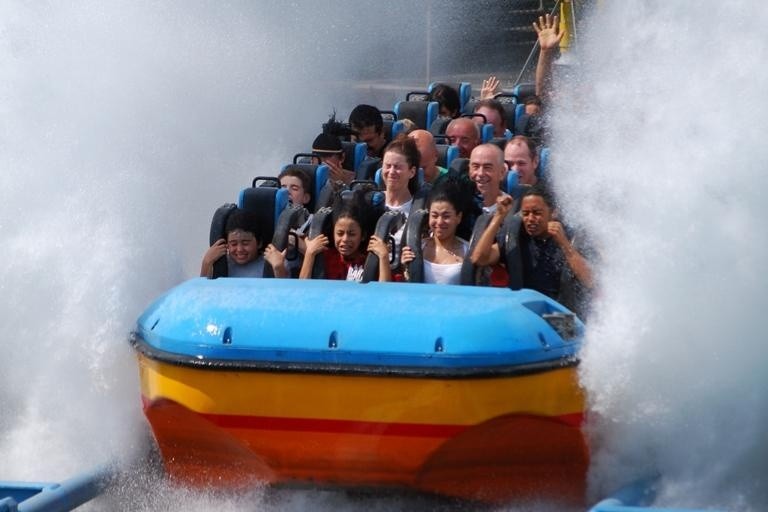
[398, 184, 489, 284]
[298, 205, 391, 281]
[198, 208, 289, 279]
[529, 12, 566, 106]
[471, 188, 597, 291]
[278, 79, 563, 271]
